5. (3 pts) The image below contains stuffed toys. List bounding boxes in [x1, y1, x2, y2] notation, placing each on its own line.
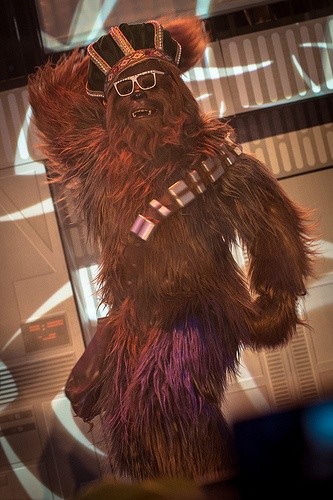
[26, 12, 318, 500]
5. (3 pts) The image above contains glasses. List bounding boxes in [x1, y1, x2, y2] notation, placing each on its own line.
[114, 70, 166, 97]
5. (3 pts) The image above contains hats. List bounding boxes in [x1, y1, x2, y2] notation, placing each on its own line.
[83, 20, 186, 101]
